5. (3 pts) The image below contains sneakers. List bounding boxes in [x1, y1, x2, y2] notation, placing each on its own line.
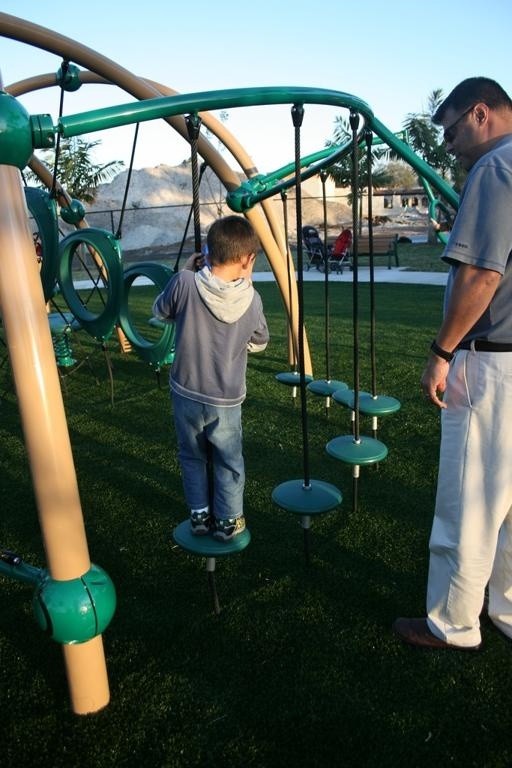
[212, 516, 246, 540]
[188, 511, 212, 537]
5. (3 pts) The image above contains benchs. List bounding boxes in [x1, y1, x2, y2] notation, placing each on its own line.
[325, 232, 400, 270]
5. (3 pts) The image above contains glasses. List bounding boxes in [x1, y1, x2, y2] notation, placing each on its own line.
[444, 102, 478, 145]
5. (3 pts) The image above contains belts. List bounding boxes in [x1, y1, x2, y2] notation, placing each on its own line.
[456, 339, 512, 353]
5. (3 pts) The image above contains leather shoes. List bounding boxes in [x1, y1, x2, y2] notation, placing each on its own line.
[479, 602, 512, 644]
[391, 615, 483, 652]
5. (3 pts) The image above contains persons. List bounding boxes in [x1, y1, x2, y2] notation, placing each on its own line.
[153, 214, 272, 541]
[391, 75, 512, 652]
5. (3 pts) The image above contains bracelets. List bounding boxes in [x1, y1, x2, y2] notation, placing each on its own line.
[429, 338, 455, 363]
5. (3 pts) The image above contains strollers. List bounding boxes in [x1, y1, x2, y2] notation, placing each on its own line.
[325, 228, 356, 276]
[301, 221, 329, 273]
